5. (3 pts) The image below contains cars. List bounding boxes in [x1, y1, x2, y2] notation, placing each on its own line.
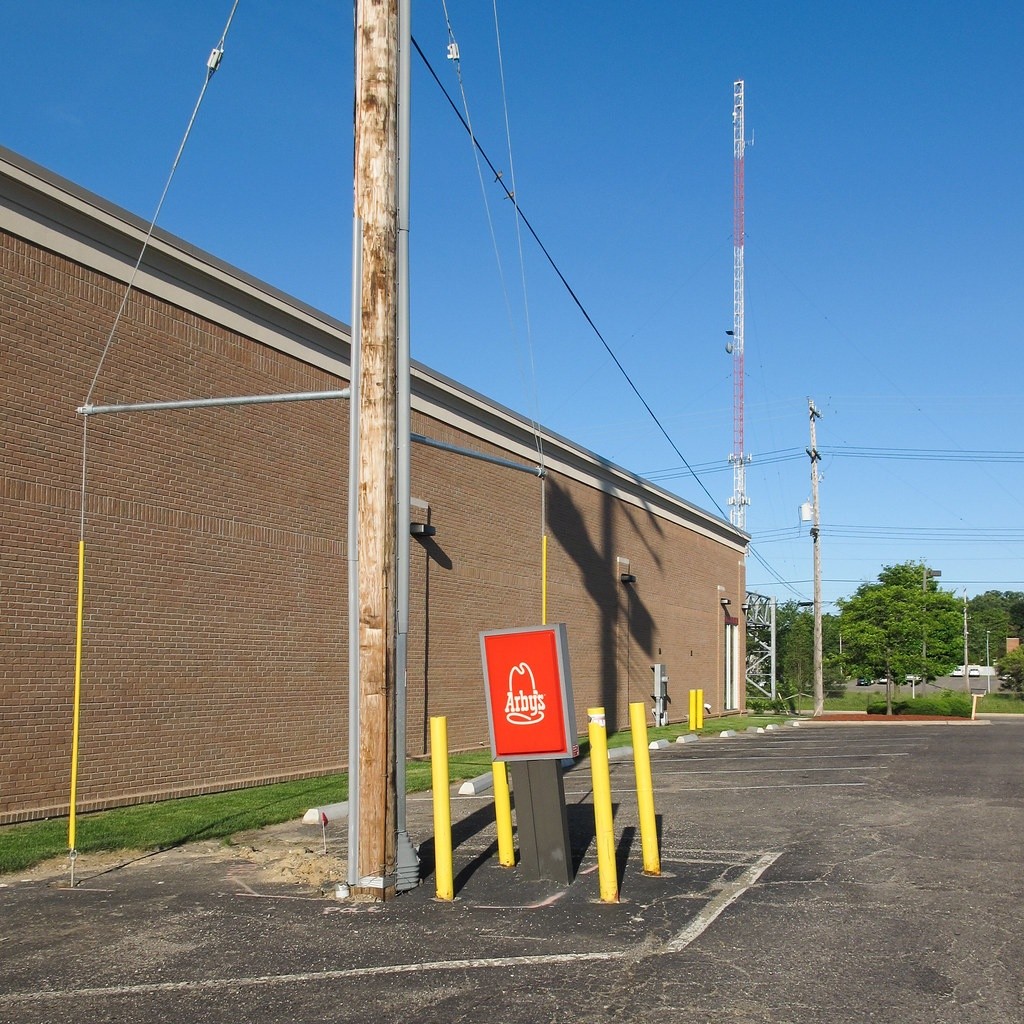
[954, 668, 964, 677]
[878, 678, 887, 684]
[968, 668, 980, 677]
[857, 677, 871, 686]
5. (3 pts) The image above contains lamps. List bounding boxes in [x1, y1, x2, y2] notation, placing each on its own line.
[621, 573, 636, 583]
[721, 598, 732, 605]
[742, 603, 751, 609]
[410, 522, 436, 537]
